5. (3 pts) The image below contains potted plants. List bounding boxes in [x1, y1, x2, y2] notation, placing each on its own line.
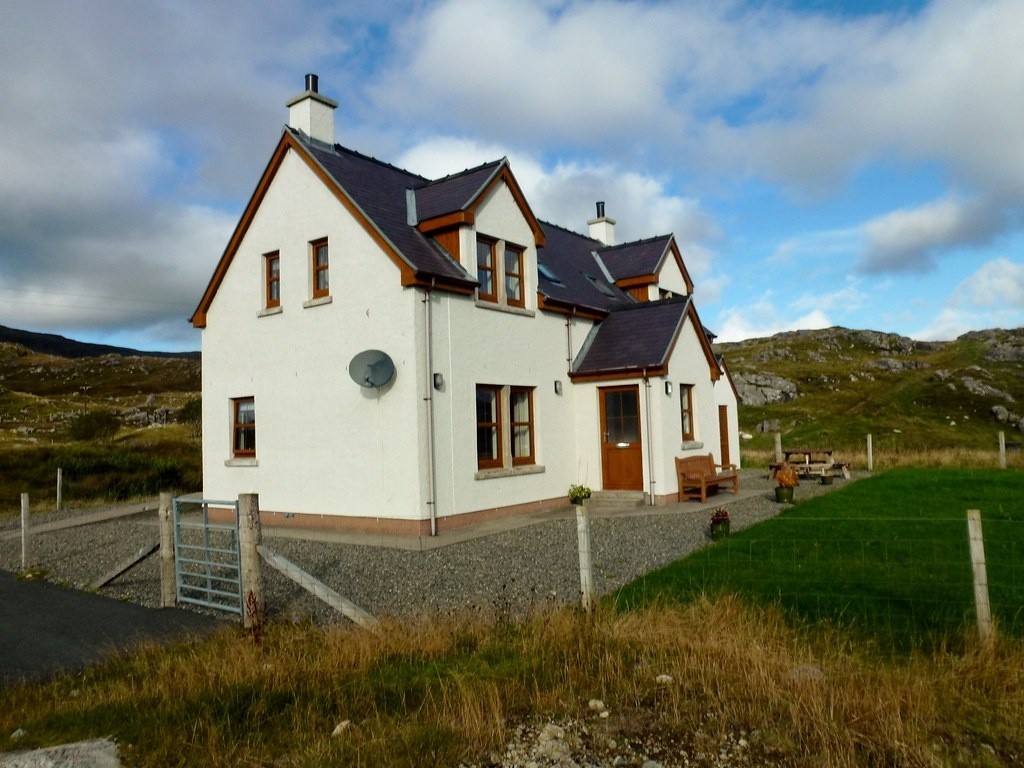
[775, 462, 800, 503]
[710, 508, 730, 541]
[568, 485, 592, 506]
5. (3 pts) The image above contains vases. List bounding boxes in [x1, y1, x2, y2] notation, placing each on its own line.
[820, 475, 834, 484]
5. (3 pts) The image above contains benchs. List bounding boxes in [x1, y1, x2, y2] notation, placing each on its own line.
[675, 453, 739, 504]
[769, 461, 851, 481]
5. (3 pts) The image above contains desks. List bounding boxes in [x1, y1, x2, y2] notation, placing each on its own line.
[782, 451, 834, 478]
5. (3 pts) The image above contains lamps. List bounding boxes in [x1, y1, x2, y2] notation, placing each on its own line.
[665, 381, 673, 395]
[433, 373, 443, 390]
[554, 381, 563, 394]
